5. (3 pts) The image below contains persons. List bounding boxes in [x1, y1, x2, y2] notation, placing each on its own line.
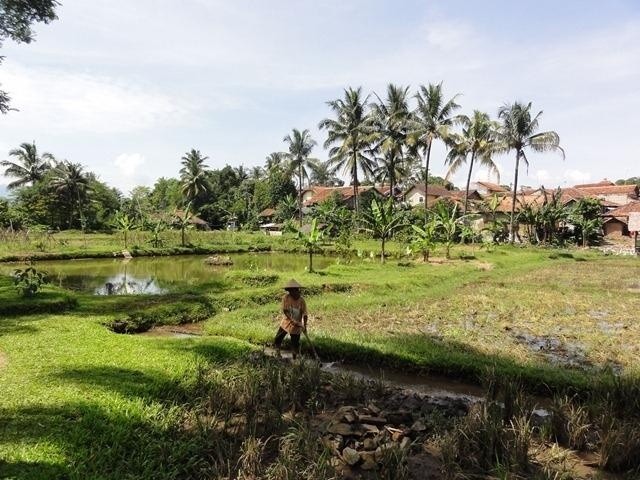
[273, 277, 308, 361]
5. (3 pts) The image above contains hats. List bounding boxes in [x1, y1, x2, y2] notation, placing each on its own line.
[280, 279, 305, 289]
